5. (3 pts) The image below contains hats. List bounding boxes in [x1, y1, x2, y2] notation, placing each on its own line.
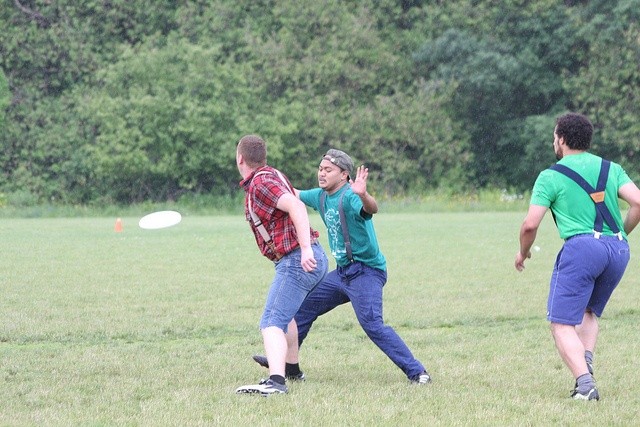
[321, 148, 355, 179]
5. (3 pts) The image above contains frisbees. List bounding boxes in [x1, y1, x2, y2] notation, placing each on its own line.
[139, 211, 181, 230]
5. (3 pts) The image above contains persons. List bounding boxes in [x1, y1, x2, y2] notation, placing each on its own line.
[515, 113, 637, 402]
[236, 135, 329, 397]
[253, 149, 429, 384]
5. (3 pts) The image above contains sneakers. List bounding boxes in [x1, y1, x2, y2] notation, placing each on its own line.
[569, 385, 600, 402]
[253, 353, 270, 368]
[234, 378, 289, 397]
[409, 369, 431, 387]
[286, 371, 306, 383]
